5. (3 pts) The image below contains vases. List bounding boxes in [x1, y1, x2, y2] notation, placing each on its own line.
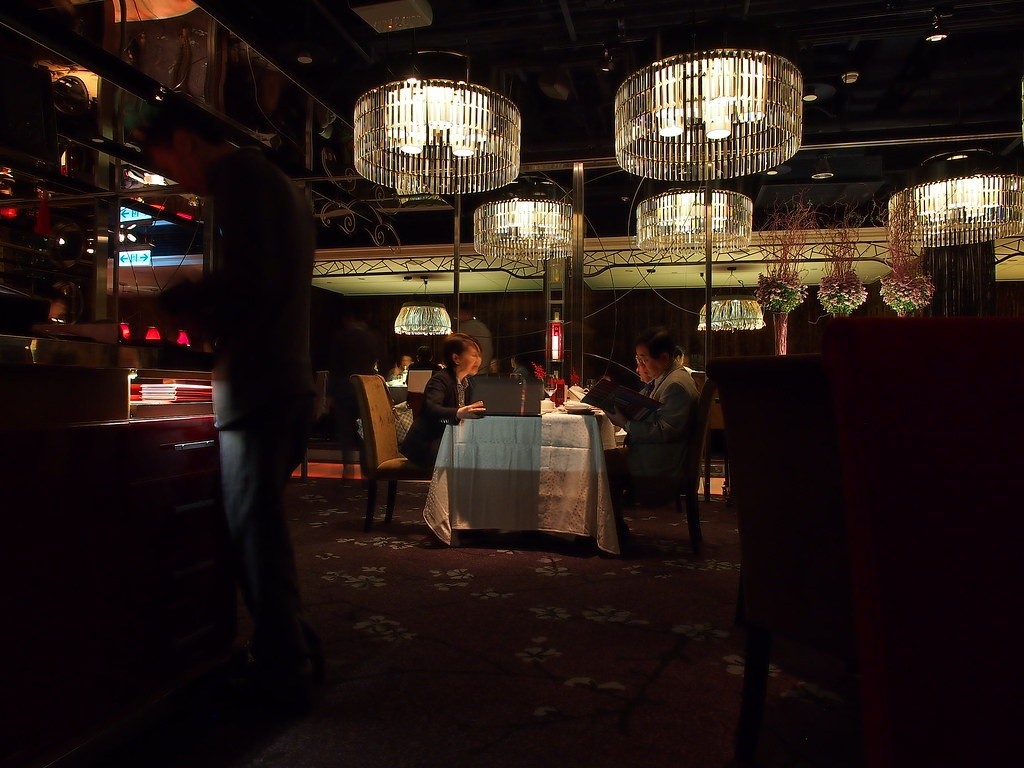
[897, 310, 925, 318]
[833, 311, 850, 318]
[774, 307, 790, 354]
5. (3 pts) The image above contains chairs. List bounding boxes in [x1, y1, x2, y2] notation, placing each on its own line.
[700, 348, 860, 763]
[605, 377, 719, 563]
[818, 311, 1024, 768]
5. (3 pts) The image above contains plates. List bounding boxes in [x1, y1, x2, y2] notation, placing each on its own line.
[541, 409, 556, 412]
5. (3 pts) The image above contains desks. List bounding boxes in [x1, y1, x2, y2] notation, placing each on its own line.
[423, 400, 622, 557]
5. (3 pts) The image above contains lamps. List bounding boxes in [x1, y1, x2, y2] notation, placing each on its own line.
[766, 166, 780, 176]
[888, 142, 1024, 250]
[472, 177, 574, 263]
[613, 0, 807, 184]
[803, 83, 818, 103]
[841, 68, 862, 84]
[697, 266, 768, 334]
[808, 151, 837, 182]
[352, 31, 524, 199]
[923, 8, 954, 45]
[393, 276, 455, 336]
[634, 185, 755, 255]
[598, 43, 616, 73]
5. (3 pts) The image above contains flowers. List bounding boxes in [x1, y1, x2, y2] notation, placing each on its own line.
[747, 193, 932, 313]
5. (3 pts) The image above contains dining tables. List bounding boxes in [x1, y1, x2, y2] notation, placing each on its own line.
[351, 373, 435, 534]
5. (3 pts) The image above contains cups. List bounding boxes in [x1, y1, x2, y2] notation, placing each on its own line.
[541, 397, 555, 409]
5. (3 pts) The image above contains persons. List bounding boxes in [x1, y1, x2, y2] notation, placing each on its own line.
[332, 296, 534, 492]
[574, 324, 702, 544]
[398, 332, 487, 542]
[123, 98, 329, 727]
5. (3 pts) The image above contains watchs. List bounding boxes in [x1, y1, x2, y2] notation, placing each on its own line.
[624, 419, 631, 433]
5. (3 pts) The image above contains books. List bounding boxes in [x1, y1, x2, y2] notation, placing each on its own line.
[568, 378, 661, 422]
[471, 380, 541, 416]
